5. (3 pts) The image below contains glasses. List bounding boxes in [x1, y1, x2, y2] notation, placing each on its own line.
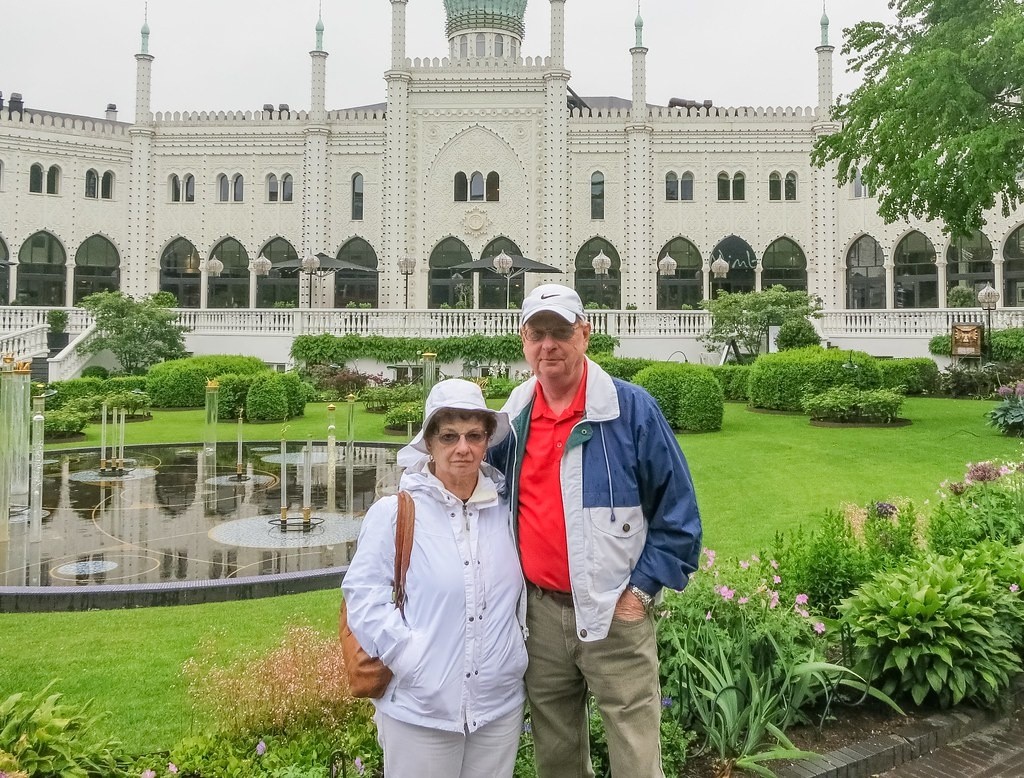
[522, 325, 581, 342]
[431, 430, 488, 444]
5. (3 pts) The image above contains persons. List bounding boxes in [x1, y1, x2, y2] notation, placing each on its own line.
[342, 378, 529, 778]
[481, 284, 702, 778]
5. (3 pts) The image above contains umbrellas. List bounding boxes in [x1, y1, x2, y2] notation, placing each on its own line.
[449, 252, 562, 309]
[271, 252, 380, 308]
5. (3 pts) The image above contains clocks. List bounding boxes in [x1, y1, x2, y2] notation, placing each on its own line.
[468, 214, 483, 231]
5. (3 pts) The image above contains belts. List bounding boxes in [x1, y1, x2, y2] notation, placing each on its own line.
[540, 587, 573, 605]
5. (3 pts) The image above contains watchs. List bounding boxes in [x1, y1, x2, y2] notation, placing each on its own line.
[626, 584, 655, 612]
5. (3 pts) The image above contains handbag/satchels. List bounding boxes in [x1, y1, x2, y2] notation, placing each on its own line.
[339, 490, 415, 699]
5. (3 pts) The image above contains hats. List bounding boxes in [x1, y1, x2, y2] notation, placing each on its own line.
[409, 379, 511, 455]
[521, 284, 586, 326]
